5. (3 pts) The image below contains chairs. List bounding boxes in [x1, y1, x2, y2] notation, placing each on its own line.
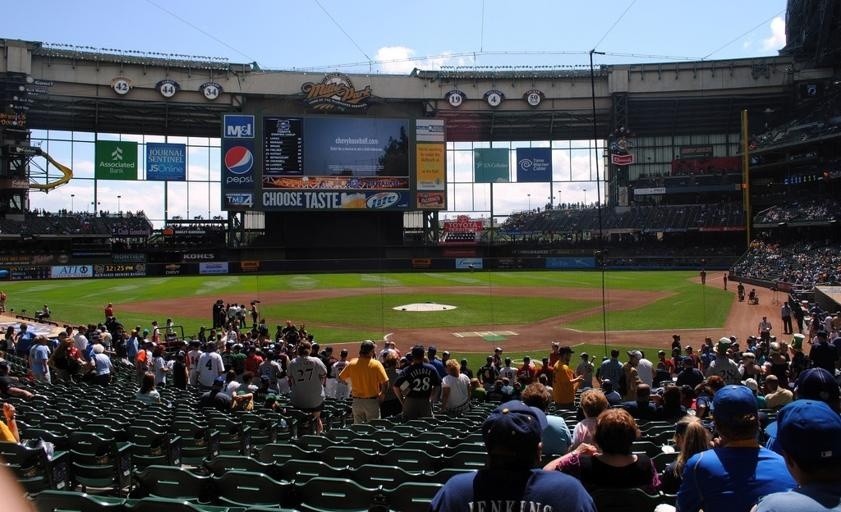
[727, 86, 841, 300]
[0, 310, 798, 511]
[0, 138, 227, 263]
[496, 169, 754, 274]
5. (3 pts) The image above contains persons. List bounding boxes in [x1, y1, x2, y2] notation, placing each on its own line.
[28, 207, 226, 250]
[2, 293, 448, 442]
[2, 465, 38, 510]
[499, 95, 840, 303]
[431, 299, 840, 512]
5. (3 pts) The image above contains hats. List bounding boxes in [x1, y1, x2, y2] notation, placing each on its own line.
[482, 400, 549, 450]
[480, 341, 589, 369]
[397, 345, 469, 367]
[740, 377, 759, 391]
[626, 350, 643, 360]
[175, 337, 350, 363]
[709, 385, 760, 426]
[765, 374, 779, 385]
[360, 340, 378, 356]
[655, 330, 789, 366]
[787, 367, 841, 403]
[763, 400, 840, 450]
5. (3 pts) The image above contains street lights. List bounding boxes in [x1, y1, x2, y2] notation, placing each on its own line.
[70, 194, 75, 212]
[583, 189, 587, 204]
[559, 190, 562, 203]
[527, 194, 531, 211]
[117, 194, 121, 213]
